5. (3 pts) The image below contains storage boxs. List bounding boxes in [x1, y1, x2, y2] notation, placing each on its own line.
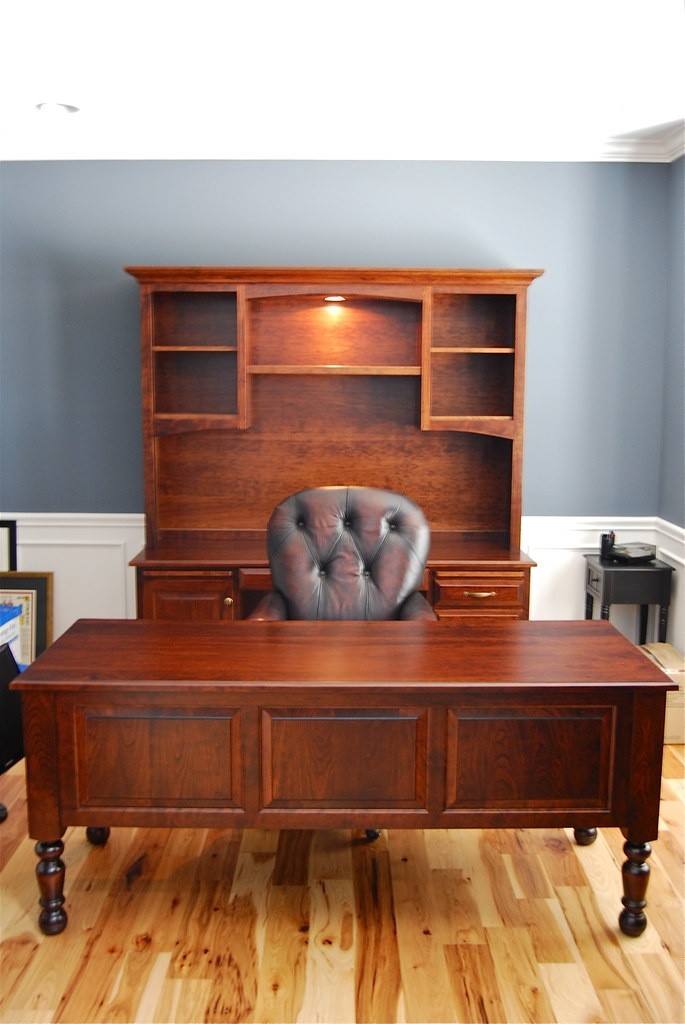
[639, 643, 685, 745]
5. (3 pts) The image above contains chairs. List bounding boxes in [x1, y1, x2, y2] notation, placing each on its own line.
[247, 486, 437, 840]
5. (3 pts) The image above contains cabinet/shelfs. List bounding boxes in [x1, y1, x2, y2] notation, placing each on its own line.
[124, 262, 545, 623]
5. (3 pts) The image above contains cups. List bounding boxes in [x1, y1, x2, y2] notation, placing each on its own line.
[601, 535, 615, 559]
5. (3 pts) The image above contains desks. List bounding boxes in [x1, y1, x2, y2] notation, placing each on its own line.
[9, 620, 680, 937]
[583, 554, 675, 643]
